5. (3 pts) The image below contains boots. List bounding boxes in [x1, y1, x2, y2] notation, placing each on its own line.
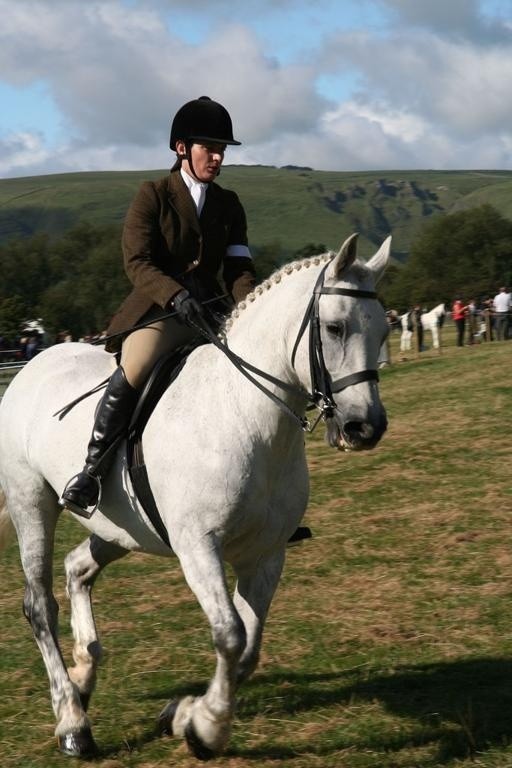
[62, 365, 139, 509]
[288, 527, 311, 543]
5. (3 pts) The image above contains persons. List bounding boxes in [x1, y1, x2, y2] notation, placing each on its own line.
[407, 303, 424, 357]
[452, 299, 468, 347]
[479, 285, 511, 342]
[465, 299, 481, 344]
[60, 94, 314, 544]
[373, 333, 390, 372]
[12, 317, 111, 360]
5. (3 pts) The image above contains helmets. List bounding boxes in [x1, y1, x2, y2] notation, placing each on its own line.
[170, 96, 242, 151]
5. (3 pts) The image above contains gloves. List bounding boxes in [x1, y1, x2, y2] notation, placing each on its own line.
[174, 289, 202, 322]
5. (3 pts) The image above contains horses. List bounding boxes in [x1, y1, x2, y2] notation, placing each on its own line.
[395, 303, 452, 353]
[0, 230, 392, 760]
